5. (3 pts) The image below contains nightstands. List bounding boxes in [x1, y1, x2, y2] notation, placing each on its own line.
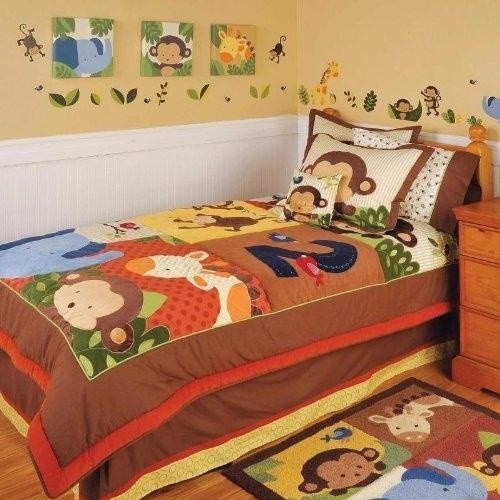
[450, 196, 500, 395]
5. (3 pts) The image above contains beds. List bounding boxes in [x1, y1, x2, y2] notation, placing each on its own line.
[0, 107, 493, 500]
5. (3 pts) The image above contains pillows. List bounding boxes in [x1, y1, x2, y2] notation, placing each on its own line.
[285, 107, 481, 236]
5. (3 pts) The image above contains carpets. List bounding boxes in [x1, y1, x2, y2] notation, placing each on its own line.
[221, 376, 500, 500]
